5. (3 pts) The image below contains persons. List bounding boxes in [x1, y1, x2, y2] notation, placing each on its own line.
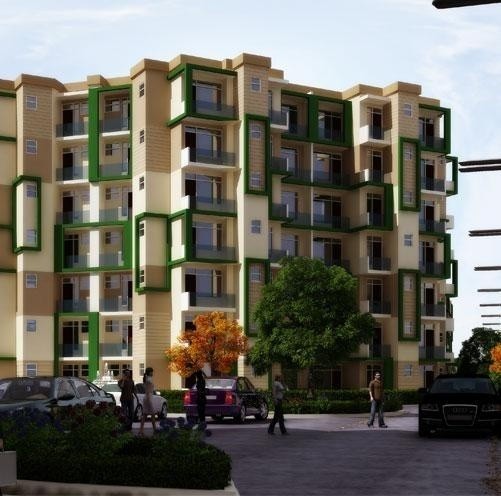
[366, 371, 388, 428]
[136, 367, 159, 435]
[267, 374, 291, 436]
[118, 368, 139, 432]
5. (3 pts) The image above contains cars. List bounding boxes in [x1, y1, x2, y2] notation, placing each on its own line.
[418, 373, 500, 438]
[0, 376, 115, 420]
[183, 375, 269, 423]
[91, 379, 168, 422]
